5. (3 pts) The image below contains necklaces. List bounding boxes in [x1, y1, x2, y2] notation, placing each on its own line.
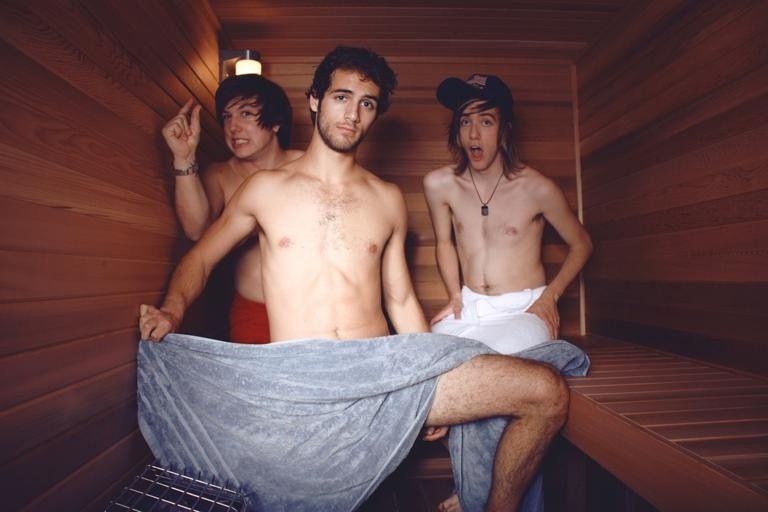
[467, 166, 506, 217]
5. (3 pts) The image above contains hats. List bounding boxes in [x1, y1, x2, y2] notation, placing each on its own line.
[437, 73, 513, 111]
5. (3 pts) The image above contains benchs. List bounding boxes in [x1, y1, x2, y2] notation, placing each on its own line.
[553, 329, 766, 510]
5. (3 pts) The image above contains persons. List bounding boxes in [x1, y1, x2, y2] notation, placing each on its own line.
[159, 74, 307, 346]
[420, 71, 598, 512]
[136, 43, 574, 512]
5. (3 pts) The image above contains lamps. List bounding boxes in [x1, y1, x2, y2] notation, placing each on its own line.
[218, 47, 263, 83]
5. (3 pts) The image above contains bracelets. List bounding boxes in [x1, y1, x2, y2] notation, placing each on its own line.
[173, 160, 200, 177]
